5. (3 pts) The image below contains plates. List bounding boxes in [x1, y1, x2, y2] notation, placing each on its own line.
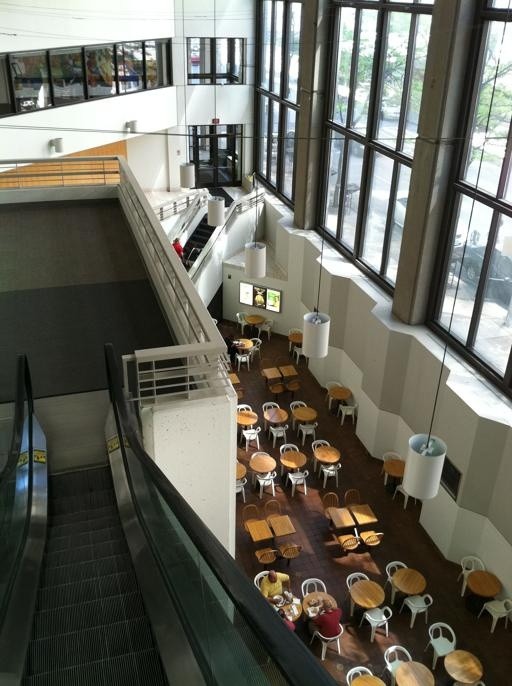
[272, 594, 284, 604]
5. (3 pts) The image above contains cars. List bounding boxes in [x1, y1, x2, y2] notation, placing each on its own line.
[332, 123, 383, 158]
[261, 124, 295, 162]
[390, 188, 512, 293]
[358, 93, 406, 122]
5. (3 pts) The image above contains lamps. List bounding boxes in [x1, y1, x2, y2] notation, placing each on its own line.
[49, 137, 64, 153]
[404, 2, 512, 498]
[125, 120, 137, 135]
[180, 0, 267, 278]
[302, 0, 342, 357]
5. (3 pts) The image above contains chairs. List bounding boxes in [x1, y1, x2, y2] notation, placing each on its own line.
[242, 490, 383, 568]
[227, 311, 309, 402]
[249, 561, 511, 686]
[234, 380, 428, 508]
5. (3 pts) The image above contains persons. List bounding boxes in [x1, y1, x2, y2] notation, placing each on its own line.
[277, 610, 297, 632]
[260, 569, 291, 603]
[307, 598, 344, 644]
[173, 237, 187, 263]
